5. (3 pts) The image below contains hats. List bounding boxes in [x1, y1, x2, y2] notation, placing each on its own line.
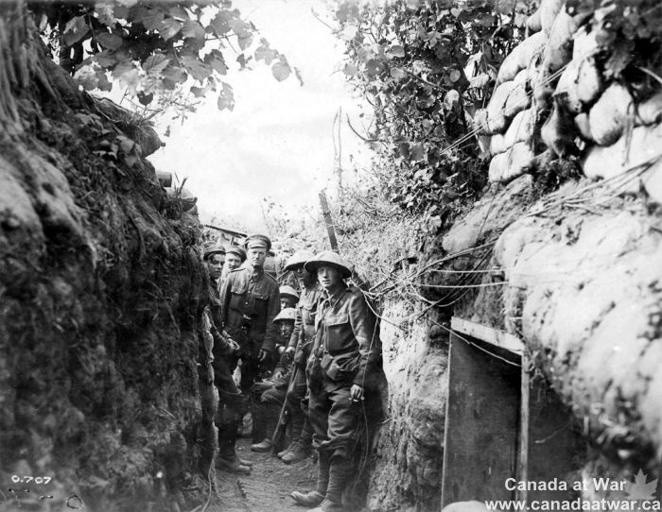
[245, 234, 272, 250]
[203, 246, 225, 259]
[273, 308, 296, 321]
[285, 250, 314, 270]
[304, 251, 352, 278]
[227, 246, 246, 260]
[278, 285, 299, 300]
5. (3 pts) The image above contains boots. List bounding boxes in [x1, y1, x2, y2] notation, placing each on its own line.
[215, 391, 311, 474]
[311, 458, 347, 512]
[291, 450, 331, 505]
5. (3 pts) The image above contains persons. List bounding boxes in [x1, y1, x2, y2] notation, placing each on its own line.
[200, 230, 378, 511]
